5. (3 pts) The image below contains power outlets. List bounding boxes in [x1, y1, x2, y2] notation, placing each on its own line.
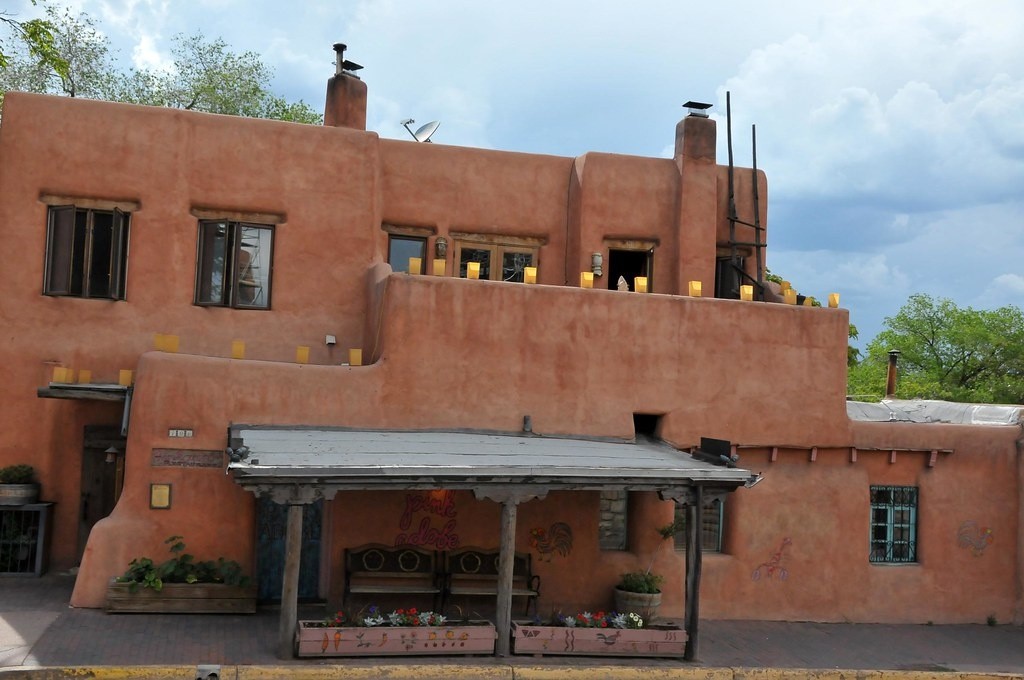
[169, 430, 193, 437]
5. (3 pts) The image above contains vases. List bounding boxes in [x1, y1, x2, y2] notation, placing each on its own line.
[510, 620, 690, 660]
[294, 620, 498, 660]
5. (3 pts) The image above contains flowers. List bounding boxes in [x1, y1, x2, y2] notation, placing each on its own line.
[323, 609, 447, 626]
[532, 610, 656, 629]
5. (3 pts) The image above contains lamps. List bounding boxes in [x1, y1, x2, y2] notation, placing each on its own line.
[105, 445, 120, 462]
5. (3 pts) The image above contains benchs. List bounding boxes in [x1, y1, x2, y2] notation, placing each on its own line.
[444, 545, 541, 620]
[343, 542, 442, 620]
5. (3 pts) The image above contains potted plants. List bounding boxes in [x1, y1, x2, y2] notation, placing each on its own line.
[105, 536, 257, 613]
[611, 517, 687, 623]
[0, 464, 42, 506]
